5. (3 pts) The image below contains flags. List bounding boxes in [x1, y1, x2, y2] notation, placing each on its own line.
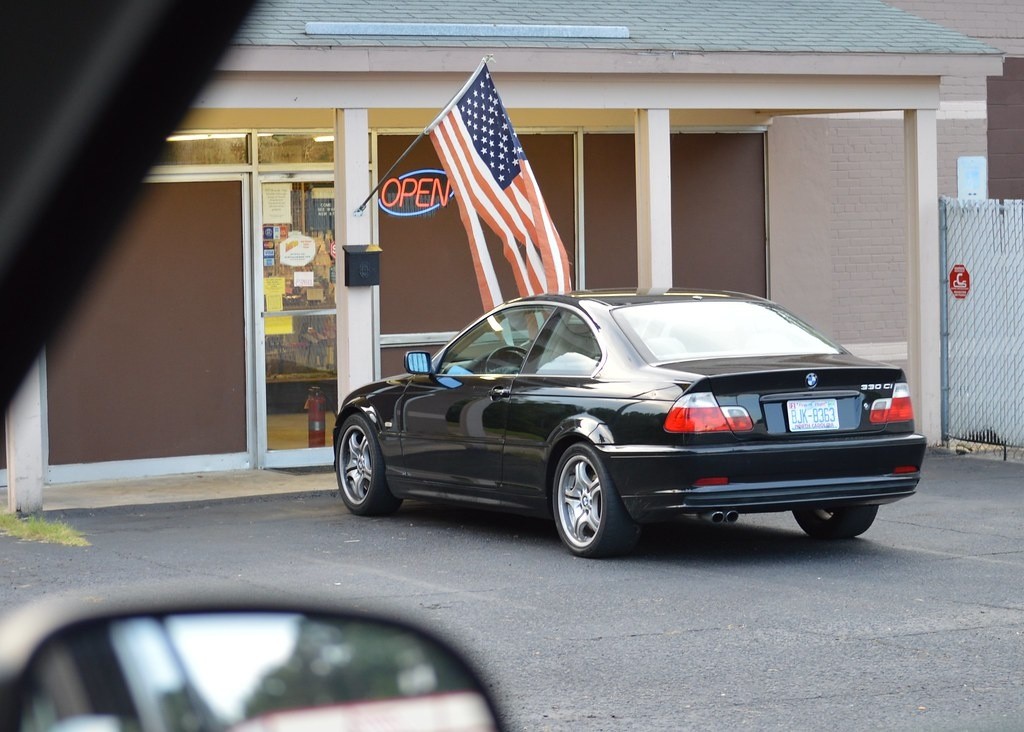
[426, 57, 573, 347]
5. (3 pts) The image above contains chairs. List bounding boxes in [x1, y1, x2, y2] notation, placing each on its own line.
[538, 313, 596, 376]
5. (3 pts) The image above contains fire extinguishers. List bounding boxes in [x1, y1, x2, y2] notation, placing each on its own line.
[305, 381, 338, 448]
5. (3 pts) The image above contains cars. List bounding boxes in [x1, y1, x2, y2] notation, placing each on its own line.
[332, 291, 928, 559]
